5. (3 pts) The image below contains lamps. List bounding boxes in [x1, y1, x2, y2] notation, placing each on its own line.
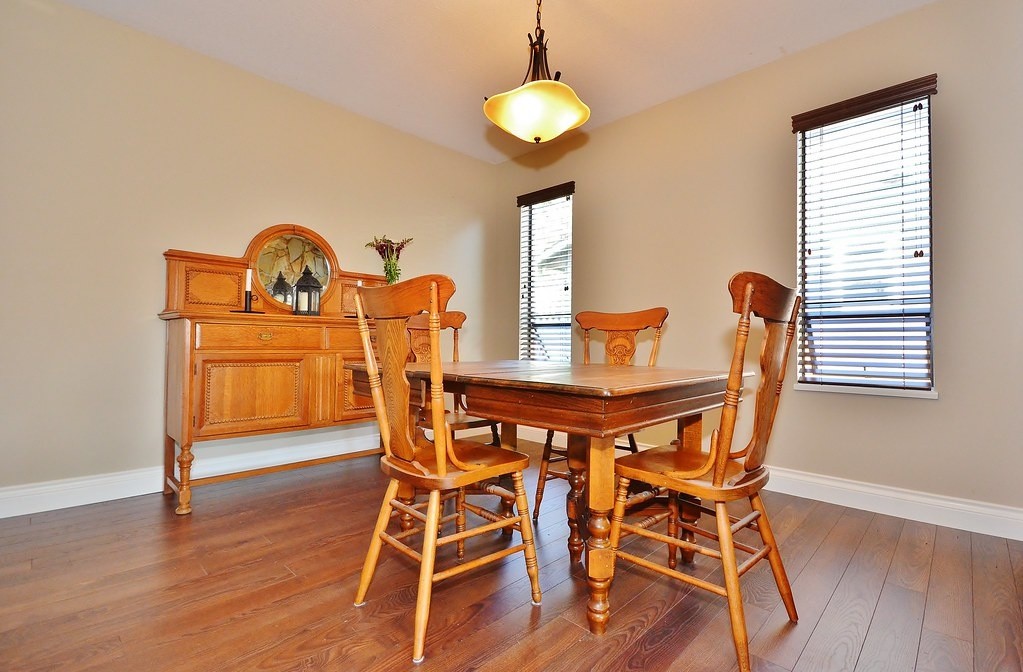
[483, 0, 591, 145]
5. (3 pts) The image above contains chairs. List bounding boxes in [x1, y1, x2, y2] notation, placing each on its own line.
[533, 307, 669, 523]
[604, 270, 802, 671]
[352, 273, 543, 664]
[379, 310, 502, 458]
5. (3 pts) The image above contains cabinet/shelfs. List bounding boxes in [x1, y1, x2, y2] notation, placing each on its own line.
[156, 223, 416, 516]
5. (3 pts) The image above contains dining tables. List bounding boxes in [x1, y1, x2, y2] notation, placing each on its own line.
[343, 360, 755, 566]
